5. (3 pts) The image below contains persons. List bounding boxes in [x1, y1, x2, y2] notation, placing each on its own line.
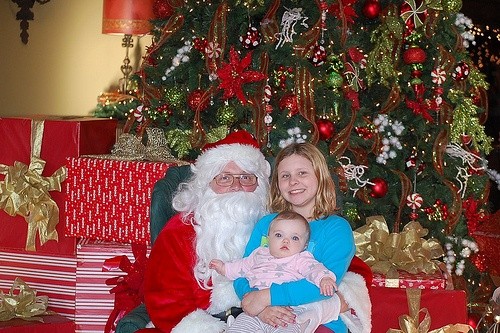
[130, 127, 373, 333]
[207, 209, 338, 333]
[234, 142, 357, 333]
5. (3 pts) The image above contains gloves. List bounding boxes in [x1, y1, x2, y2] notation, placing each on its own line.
[290, 293, 341, 332]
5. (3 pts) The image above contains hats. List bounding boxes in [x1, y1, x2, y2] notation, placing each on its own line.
[192, 128, 266, 166]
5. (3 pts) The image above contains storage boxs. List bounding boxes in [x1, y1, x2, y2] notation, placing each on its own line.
[63, 152, 180, 244]
[0, 113, 118, 257]
[370, 282, 469, 333]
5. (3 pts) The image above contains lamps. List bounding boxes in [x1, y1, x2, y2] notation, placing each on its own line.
[101, 0, 156, 100]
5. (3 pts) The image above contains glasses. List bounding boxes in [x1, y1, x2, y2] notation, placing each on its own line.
[214, 173, 259, 186]
